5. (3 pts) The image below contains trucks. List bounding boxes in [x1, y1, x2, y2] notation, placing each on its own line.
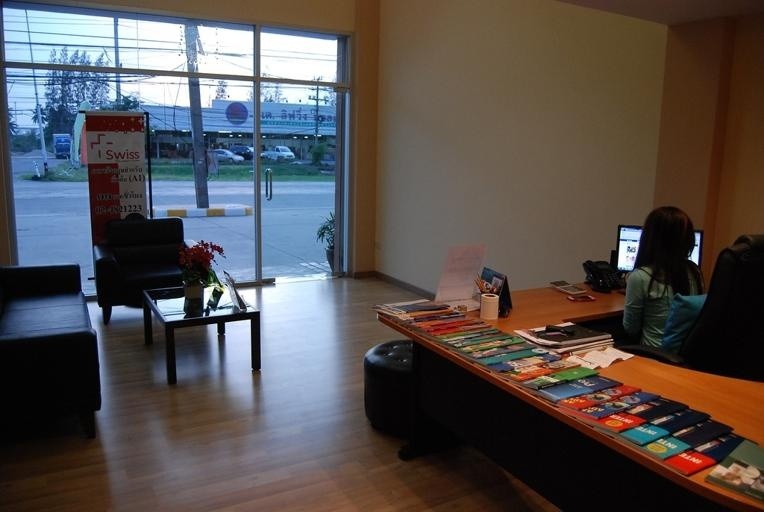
[262, 146, 295, 162]
[53, 134, 71, 160]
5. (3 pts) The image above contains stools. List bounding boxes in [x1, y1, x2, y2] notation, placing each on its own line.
[364, 335, 420, 440]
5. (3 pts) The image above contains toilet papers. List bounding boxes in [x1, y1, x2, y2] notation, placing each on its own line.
[479, 292, 500, 321]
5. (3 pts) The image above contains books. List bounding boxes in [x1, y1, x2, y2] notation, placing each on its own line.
[707, 439, 763, 499]
[620, 410, 747, 474]
[514, 322, 614, 357]
[522, 367, 689, 433]
[435, 327, 581, 381]
[371, 298, 492, 337]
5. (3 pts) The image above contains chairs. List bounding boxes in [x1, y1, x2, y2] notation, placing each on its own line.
[615, 232, 763, 380]
[90, 210, 197, 326]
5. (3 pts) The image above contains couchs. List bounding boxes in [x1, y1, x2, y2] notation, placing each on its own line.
[0, 261, 104, 448]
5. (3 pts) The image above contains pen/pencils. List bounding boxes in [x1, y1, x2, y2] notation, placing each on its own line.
[474, 271, 486, 293]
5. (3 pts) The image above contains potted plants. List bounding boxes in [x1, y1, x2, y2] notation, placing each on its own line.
[309, 209, 335, 276]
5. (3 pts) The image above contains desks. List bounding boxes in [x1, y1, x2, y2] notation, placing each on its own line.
[377, 273, 764, 512]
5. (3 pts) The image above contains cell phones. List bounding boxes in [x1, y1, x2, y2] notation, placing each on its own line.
[567, 295, 595, 301]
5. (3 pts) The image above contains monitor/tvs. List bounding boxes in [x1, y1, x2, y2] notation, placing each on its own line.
[616, 224, 704, 272]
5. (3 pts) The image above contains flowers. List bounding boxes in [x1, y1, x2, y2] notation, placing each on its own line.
[176, 238, 226, 281]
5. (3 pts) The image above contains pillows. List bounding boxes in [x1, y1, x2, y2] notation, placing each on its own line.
[659, 290, 710, 352]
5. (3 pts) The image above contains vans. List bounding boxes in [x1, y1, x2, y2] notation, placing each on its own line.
[229, 146, 267, 160]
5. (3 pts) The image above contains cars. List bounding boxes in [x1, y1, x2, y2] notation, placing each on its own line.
[206, 149, 244, 165]
[318, 152, 336, 172]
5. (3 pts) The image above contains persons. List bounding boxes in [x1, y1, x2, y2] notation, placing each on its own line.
[623, 205, 706, 365]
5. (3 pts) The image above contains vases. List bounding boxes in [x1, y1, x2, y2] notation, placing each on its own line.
[182, 280, 206, 300]
